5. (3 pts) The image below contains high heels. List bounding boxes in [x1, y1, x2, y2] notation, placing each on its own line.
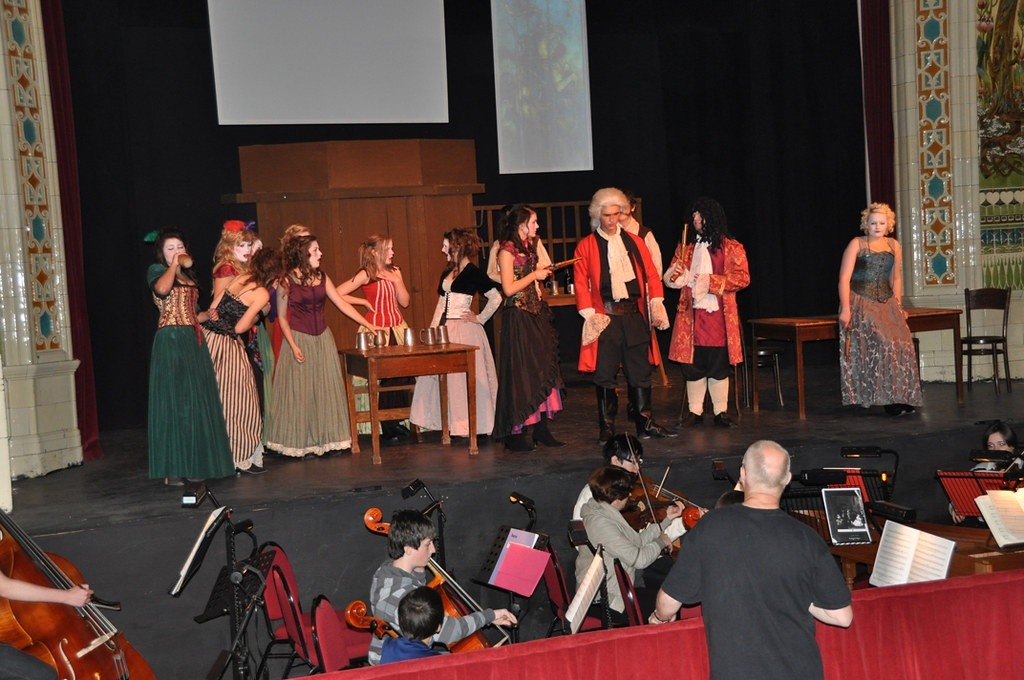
[504, 434, 533, 451]
[532, 420, 567, 448]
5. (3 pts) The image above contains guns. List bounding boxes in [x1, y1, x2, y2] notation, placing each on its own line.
[843, 318, 852, 360]
[542, 257, 583, 273]
[669, 223, 689, 282]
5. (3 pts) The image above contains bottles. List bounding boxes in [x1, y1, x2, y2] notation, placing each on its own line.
[564, 268, 572, 294]
[178, 255, 193, 268]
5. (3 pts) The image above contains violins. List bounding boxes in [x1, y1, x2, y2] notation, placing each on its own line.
[998, 447, 1024, 472]
[619, 492, 678, 523]
[620, 472, 703, 560]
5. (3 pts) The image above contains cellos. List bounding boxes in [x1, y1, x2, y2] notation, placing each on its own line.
[364, 507, 512, 654]
[345, 601, 400, 639]
[680, 503, 702, 530]
[0, 505, 157, 680]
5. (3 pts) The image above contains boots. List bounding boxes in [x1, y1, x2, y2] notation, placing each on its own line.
[633, 410, 679, 439]
[599, 415, 615, 445]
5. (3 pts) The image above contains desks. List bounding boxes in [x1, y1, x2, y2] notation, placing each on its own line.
[338, 343, 480, 464]
[791, 509, 999, 591]
[748, 307, 963, 419]
[492, 287, 576, 380]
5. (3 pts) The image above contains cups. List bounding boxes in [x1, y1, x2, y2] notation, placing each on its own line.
[404, 328, 415, 347]
[358, 333, 369, 352]
[425, 328, 435, 346]
[550, 281, 560, 296]
[436, 326, 449, 344]
[374, 330, 386, 349]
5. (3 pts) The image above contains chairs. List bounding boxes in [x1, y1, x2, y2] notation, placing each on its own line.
[541, 532, 644, 638]
[256, 539, 373, 680]
[678, 316, 784, 417]
[961, 286, 1012, 398]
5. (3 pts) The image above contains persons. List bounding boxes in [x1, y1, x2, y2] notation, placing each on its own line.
[0, 570, 95, 680]
[839, 203, 924, 416]
[147, 221, 409, 486]
[413, 188, 750, 452]
[949, 421, 1024, 523]
[366, 434, 708, 673]
[649, 440, 853, 680]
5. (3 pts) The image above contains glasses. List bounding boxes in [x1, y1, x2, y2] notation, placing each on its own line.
[626, 458, 644, 465]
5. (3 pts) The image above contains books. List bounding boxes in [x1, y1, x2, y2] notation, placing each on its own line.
[820, 488, 873, 547]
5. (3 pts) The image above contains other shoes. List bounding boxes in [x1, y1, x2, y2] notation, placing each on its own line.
[885, 403, 916, 418]
[695, 413, 704, 427]
[714, 411, 738, 429]
[247, 463, 268, 474]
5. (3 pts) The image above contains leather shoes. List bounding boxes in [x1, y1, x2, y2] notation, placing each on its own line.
[380, 429, 401, 445]
[394, 425, 411, 440]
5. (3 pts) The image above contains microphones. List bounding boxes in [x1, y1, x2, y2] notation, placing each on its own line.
[231, 572, 255, 600]
